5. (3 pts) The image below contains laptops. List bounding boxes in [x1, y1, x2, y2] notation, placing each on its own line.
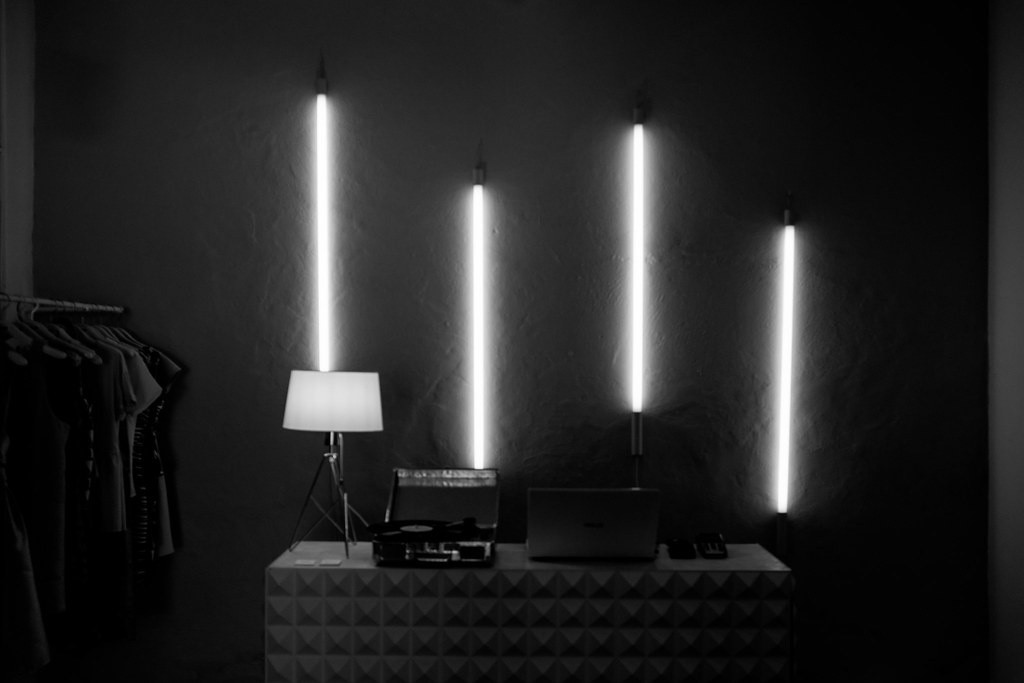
[525, 486, 661, 562]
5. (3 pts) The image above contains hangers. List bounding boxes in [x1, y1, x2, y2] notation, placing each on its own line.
[0, 292, 154, 366]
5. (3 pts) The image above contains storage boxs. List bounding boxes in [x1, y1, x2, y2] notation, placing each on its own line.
[371, 469, 501, 568]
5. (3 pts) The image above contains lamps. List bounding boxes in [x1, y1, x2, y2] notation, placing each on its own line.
[472, 165, 486, 469]
[633, 104, 643, 457]
[316, 75, 330, 372]
[282, 370, 383, 559]
[777, 210, 796, 530]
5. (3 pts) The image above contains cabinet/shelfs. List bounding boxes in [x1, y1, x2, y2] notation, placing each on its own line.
[264, 542, 793, 683]
